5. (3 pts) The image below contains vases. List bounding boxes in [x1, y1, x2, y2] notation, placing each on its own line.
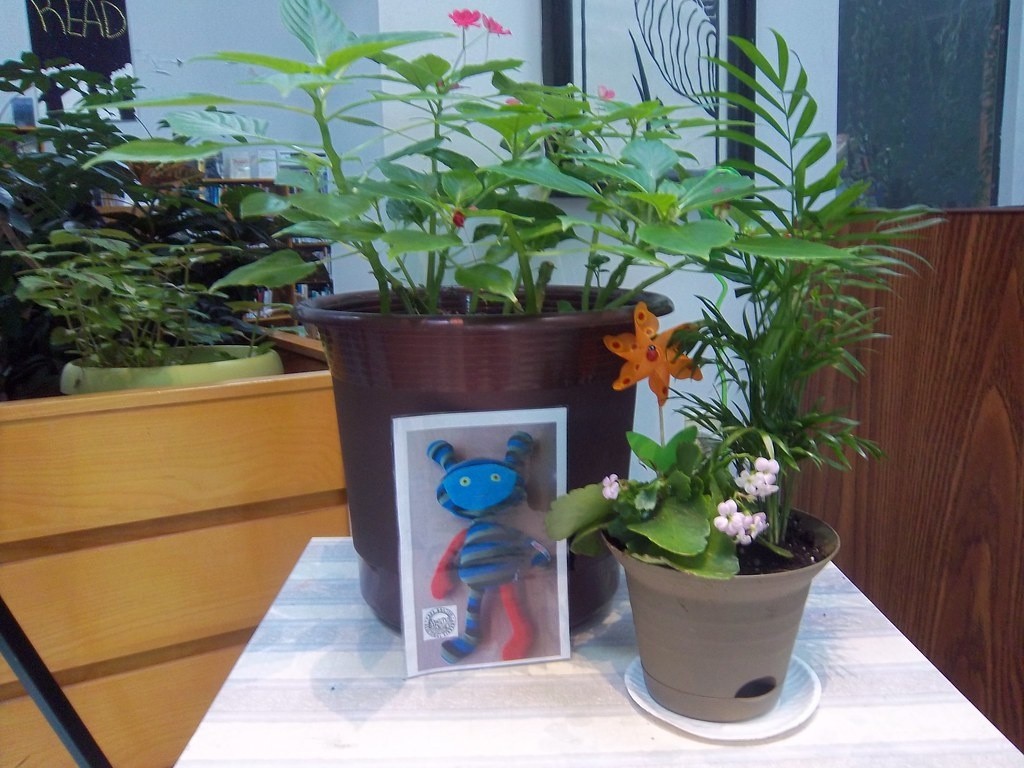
[601, 507, 842, 724]
[291, 284, 675, 639]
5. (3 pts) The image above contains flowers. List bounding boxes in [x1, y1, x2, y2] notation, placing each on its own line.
[542, 16, 951, 581]
[83, 0, 756, 319]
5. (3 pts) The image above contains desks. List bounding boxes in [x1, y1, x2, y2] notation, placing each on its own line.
[165, 536, 1024, 768]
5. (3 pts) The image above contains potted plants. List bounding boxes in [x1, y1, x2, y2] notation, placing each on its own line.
[16, 205, 283, 401]
